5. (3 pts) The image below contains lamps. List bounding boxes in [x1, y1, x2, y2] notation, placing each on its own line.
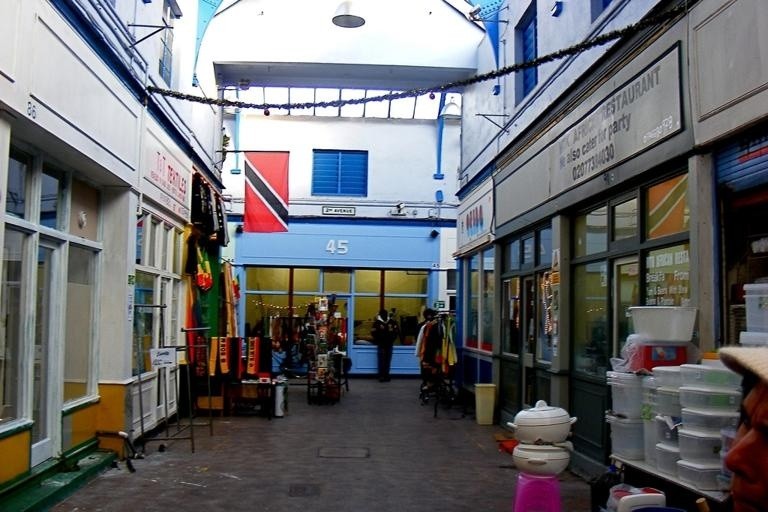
[331, 2, 365, 28]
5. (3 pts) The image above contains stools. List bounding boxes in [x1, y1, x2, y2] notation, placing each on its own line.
[511, 472, 562, 512]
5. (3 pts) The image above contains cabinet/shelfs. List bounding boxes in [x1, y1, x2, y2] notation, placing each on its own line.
[306, 295, 343, 406]
[196, 356, 288, 418]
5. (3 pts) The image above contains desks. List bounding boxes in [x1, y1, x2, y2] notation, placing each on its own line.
[608, 452, 734, 511]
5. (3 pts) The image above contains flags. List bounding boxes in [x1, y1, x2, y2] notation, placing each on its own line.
[242, 150, 291, 233]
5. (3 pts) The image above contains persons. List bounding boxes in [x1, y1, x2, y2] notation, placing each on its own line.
[714, 343, 768, 511]
[386, 307, 401, 332]
[245, 322, 253, 337]
[413, 308, 438, 342]
[371, 309, 399, 382]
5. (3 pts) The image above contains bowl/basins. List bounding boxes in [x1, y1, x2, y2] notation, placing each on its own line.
[628, 306, 699, 342]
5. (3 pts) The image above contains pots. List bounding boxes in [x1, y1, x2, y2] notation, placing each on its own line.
[512, 444, 571, 476]
[507, 400, 577, 444]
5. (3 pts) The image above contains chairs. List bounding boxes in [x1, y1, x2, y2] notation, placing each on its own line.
[340, 358, 352, 392]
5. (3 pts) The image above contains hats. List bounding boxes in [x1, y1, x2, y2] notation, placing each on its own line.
[717, 345, 768, 382]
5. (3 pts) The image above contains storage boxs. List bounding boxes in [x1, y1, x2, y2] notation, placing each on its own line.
[605, 281, 768, 490]
